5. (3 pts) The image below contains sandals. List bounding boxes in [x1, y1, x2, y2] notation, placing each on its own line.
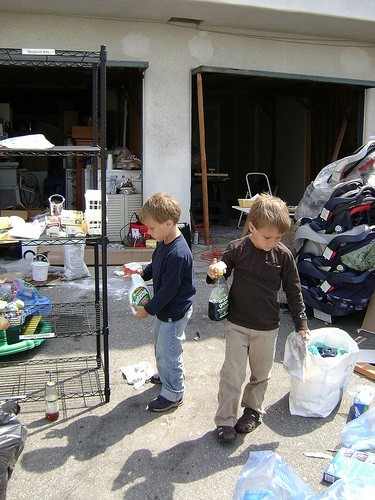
[235, 408, 259, 432]
[217, 425, 236, 442]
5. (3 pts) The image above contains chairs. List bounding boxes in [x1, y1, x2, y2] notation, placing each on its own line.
[238, 172, 273, 229]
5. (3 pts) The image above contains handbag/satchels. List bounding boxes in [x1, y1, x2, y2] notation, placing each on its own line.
[289, 327, 359, 416]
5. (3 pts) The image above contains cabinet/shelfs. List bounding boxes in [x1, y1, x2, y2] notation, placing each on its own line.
[99, 170, 141, 195]
[0, 44, 143, 403]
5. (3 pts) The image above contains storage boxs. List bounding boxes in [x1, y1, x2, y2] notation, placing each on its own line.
[322, 447, 375, 483]
[314, 308, 340, 324]
[71, 126, 101, 140]
[237, 199, 253, 207]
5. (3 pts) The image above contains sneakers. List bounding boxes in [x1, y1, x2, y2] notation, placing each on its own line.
[151, 373, 186, 384]
[148, 395, 183, 411]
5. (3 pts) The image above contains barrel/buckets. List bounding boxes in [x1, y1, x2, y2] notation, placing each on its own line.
[91, 154, 113, 170]
[83, 189, 109, 234]
[31, 253, 50, 282]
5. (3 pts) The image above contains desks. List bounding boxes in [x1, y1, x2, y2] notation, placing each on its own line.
[232, 206, 296, 218]
[192, 176, 231, 226]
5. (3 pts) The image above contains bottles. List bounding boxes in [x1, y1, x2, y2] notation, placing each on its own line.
[352, 384, 371, 418]
[193, 227, 200, 244]
[0, 305, 21, 345]
[43, 382, 59, 421]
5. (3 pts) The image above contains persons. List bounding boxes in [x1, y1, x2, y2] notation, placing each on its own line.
[125, 193, 195, 412]
[206, 194, 312, 442]
